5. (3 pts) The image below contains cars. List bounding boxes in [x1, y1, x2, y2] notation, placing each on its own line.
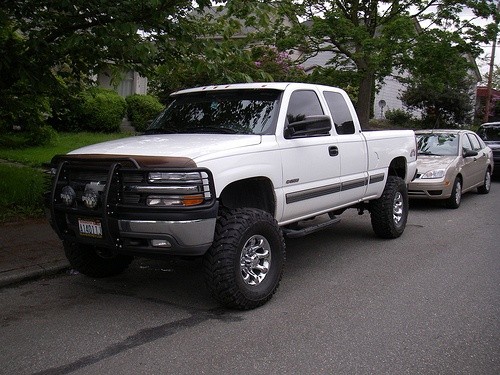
[475, 122, 500, 178]
[407, 129, 494, 209]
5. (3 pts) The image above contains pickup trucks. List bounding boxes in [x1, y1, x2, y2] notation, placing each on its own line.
[42, 82, 418, 308]
